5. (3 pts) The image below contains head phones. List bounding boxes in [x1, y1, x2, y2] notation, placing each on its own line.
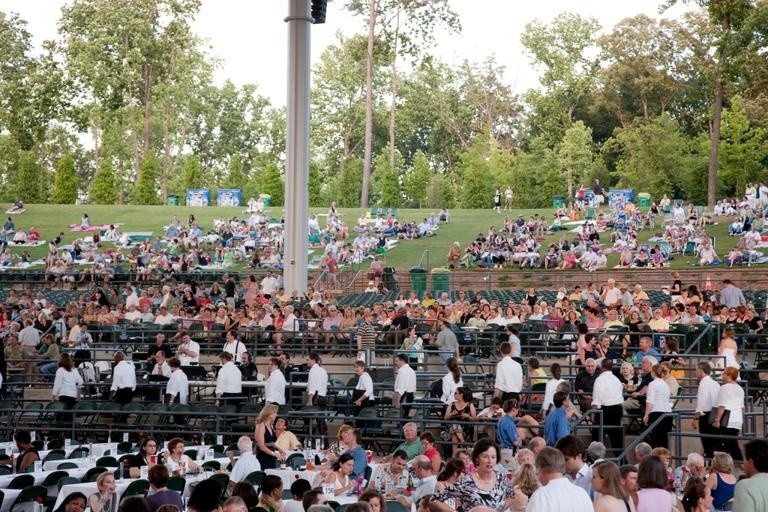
[243, 352, 252, 361]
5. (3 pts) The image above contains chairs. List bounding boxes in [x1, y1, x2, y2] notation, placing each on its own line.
[379, 207, 389, 219]
[391, 208, 397, 218]
[0, 269, 768, 512]
[370, 207, 378, 219]
[665, 199, 682, 214]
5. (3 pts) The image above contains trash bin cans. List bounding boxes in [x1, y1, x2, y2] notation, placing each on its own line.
[553, 196, 563, 208]
[638, 192, 650, 207]
[410, 269, 426, 291]
[260, 194, 271, 206]
[168, 195, 178, 206]
[432, 268, 449, 290]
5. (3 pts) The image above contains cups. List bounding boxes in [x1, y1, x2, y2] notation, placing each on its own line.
[141, 466, 148, 479]
[205, 449, 214, 463]
[34, 460, 42, 475]
[6, 447, 12, 455]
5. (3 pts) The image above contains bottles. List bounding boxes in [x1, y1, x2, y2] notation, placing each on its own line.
[357, 472, 362, 496]
[79, 451, 87, 470]
[373, 473, 414, 499]
[148, 454, 186, 479]
[280, 438, 320, 471]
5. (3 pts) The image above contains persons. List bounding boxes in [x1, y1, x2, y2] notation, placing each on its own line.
[1, 177, 767, 512]
